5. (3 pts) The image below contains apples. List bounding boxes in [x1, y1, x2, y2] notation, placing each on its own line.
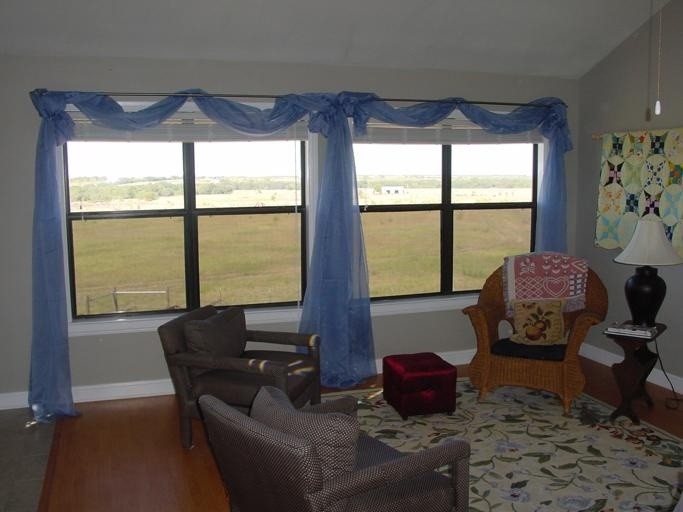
[526, 327, 542, 340]
[535, 320, 546, 331]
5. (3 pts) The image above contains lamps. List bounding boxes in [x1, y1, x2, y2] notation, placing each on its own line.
[612, 219, 682, 328]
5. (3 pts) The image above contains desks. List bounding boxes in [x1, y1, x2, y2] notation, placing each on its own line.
[602, 320, 668, 425]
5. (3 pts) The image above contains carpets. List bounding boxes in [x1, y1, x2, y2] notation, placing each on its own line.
[0, 407, 56, 512]
[321, 375, 682, 512]
